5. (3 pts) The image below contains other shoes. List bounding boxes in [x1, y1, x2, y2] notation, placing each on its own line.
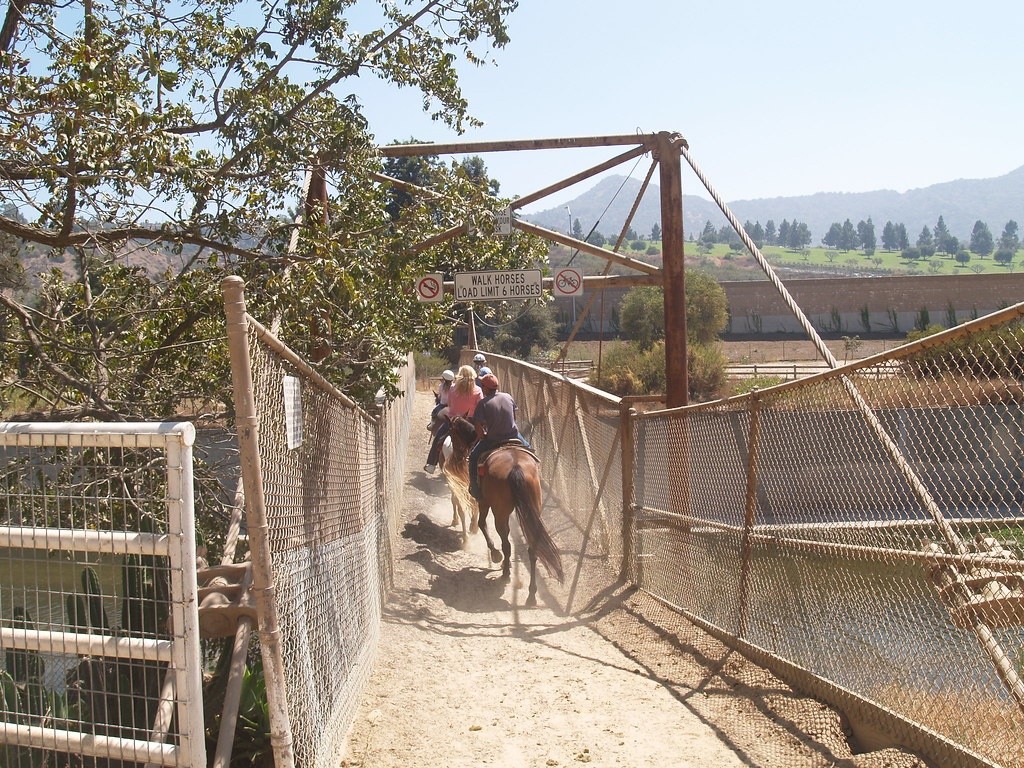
[469, 485, 479, 497]
[424, 463, 436, 474]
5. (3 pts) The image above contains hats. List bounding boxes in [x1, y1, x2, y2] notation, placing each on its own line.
[442, 370, 454, 381]
[478, 374, 499, 389]
[478, 367, 492, 376]
[472, 353, 485, 362]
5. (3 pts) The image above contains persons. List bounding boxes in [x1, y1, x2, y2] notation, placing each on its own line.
[468, 373, 531, 498]
[424, 353, 493, 474]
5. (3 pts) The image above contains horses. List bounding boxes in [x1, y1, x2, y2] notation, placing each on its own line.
[427, 389, 566, 608]
